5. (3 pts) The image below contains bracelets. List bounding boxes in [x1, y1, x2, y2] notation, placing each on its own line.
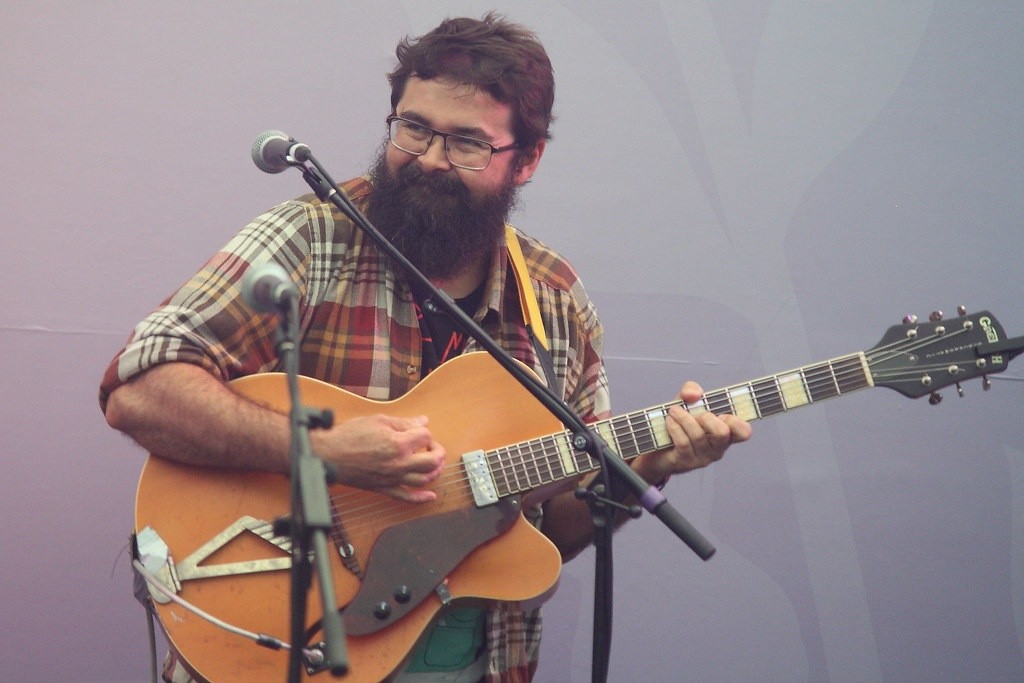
[627, 457, 670, 499]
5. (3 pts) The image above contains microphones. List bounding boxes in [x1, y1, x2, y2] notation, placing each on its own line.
[251, 130, 312, 174]
[242, 261, 301, 312]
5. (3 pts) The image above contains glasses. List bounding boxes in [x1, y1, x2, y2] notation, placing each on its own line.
[386, 111, 525, 170]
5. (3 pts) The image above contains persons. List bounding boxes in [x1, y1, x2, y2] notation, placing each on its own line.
[97, 11, 752, 683]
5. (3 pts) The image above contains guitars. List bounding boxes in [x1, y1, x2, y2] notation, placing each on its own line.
[135, 304, 1024, 683]
